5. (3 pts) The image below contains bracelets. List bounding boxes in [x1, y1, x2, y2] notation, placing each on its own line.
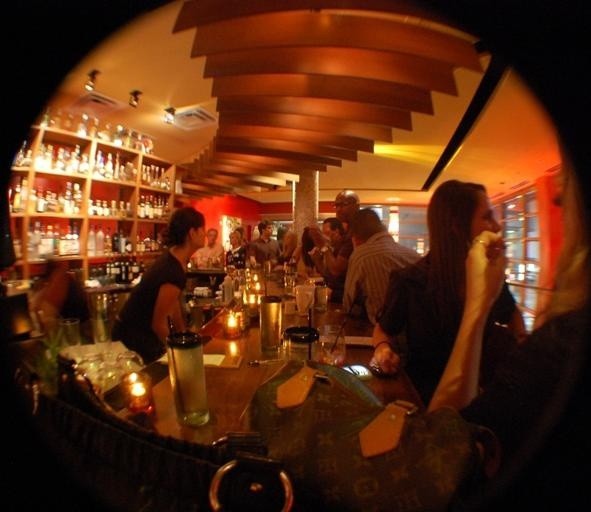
[373, 340, 391, 351]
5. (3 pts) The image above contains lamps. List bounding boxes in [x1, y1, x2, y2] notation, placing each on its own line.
[129, 91, 142, 107]
[163, 107, 177, 124]
[84, 68, 100, 93]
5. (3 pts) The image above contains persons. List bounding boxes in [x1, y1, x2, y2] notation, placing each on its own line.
[340, 208, 422, 324]
[107, 206, 208, 362]
[195, 217, 356, 301]
[425, 164, 591, 512]
[371, 179, 527, 402]
[309, 186, 361, 279]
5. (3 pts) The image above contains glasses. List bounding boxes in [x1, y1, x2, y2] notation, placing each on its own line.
[330, 200, 355, 209]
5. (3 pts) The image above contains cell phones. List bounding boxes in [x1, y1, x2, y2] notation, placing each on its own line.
[340, 364, 373, 381]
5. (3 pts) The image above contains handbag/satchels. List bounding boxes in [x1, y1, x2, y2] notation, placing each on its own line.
[211, 358, 502, 510]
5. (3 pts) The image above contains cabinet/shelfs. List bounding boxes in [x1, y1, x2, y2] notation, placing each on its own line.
[1, 124, 177, 341]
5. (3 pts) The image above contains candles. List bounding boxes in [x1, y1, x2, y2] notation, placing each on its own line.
[122, 268, 262, 416]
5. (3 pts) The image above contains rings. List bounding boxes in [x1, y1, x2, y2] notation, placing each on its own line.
[476, 239, 489, 248]
[372, 358, 385, 375]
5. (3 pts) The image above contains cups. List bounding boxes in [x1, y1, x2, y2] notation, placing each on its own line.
[295, 282, 329, 318]
[260, 295, 283, 357]
[166, 332, 210, 427]
[315, 325, 346, 369]
[282, 325, 322, 362]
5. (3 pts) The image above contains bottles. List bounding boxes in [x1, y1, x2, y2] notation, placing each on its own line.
[224, 272, 232, 305]
[10, 106, 171, 318]
